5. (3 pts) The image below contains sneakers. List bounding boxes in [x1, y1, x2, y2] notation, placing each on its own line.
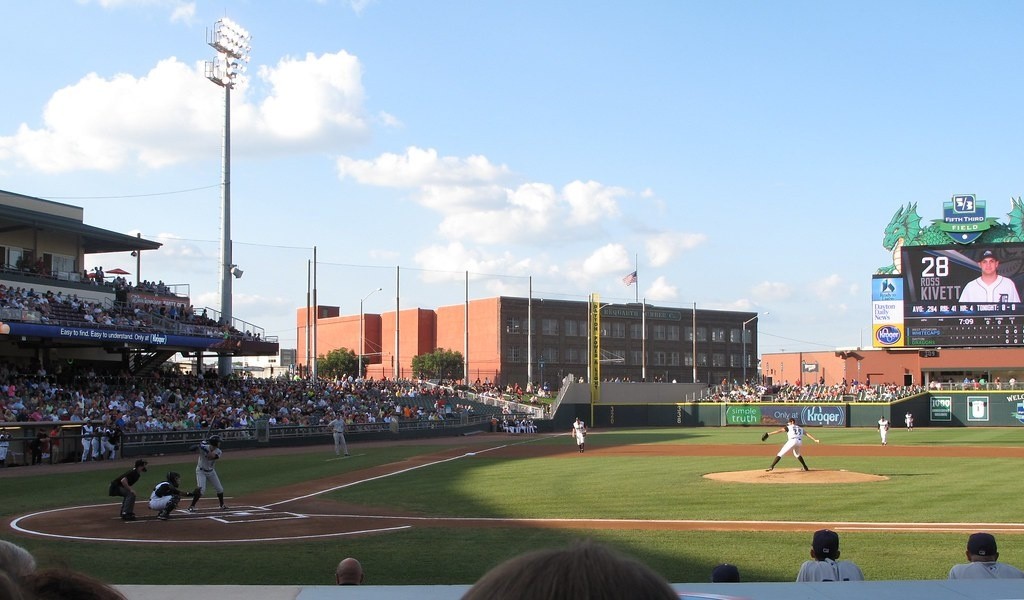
[219, 504, 230, 510]
[186, 506, 194, 513]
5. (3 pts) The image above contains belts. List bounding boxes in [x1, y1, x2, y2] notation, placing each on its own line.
[200, 468, 213, 472]
[338, 432, 343, 433]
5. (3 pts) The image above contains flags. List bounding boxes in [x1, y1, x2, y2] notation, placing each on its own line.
[623, 271, 636, 285]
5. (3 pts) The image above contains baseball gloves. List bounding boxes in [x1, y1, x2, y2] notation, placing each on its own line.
[582, 432, 587, 437]
[884, 426, 889, 431]
[761, 432, 769, 441]
[194, 487, 203, 499]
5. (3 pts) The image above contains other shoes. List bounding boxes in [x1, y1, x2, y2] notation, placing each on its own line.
[157, 511, 168, 520]
[120, 511, 137, 521]
[800, 468, 809, 471]
[765, 469, 773, 472]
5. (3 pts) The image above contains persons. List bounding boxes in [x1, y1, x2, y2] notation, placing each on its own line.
[948, 533, 1024, 580]
[958, 250, 1019, 303]
[572, 417, 587, 453]
[334, 558, 364, 586]
[762, 418, 819, 471]
[460, 542, 681, 600]
[711, 564, 739, 582]
[877, 415, 891, 444]
[82, 267, 176, 291]
[995, 377, 1002, 390]
[604, 377, 630, 382]
[1009, 376, 1018, 390]
[904, 411, 913, 431]
[654, 377, 662, 383]
[17, 255, 49, 278]
[707, 377, 943, 402]
[0, 541, 127, 600]
[0, 364, 552, 468]
[579, 376, 584, 383]
[0, 284, 260, 342]
[956, 378, 990, 390]
[109, 459, 148, 521]
[188, 435, 231, 513]
[795, 529, 865, 582]
[148, 472, 202, 520]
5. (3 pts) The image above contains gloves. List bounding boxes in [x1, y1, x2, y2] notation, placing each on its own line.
[199, 440, 209, 454]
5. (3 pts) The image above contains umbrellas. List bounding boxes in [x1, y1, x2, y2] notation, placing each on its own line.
[86, 274, 97, 279]
[105, 269, 131, 274]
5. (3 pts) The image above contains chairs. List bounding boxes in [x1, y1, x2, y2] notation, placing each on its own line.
[394, 397, 525, 422]
[48, 304, 87, 327]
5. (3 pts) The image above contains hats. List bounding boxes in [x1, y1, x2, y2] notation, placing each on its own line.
[811, 530, 839, 554]
[87, 419, 92, 422]
[712, 564, 739, 583]
[967, 533, 997, 556]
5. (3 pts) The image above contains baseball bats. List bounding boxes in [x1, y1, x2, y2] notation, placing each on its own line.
[200, 415, 217, 450]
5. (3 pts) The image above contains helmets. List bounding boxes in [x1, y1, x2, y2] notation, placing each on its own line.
[167, 472, 181, 488]
[134, 458, 148, 472]
[208, 435, 222, 448]
